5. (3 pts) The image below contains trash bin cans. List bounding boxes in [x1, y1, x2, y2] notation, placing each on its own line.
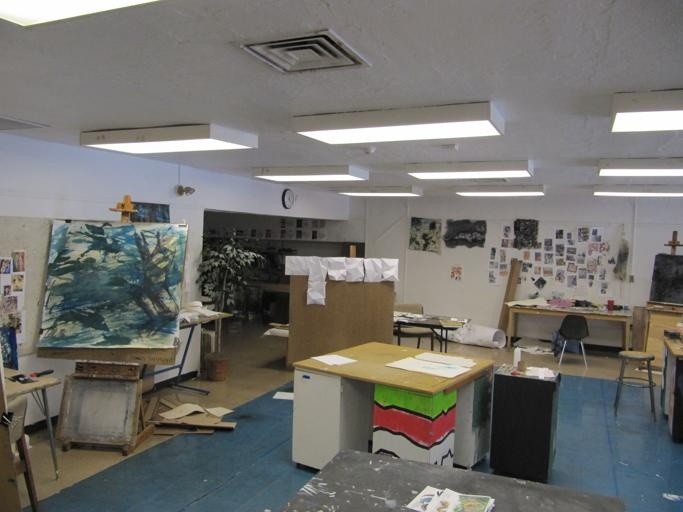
[249, 343, 271, 366]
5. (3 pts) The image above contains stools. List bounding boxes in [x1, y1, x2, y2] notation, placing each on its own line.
[610, 350, 660, 425]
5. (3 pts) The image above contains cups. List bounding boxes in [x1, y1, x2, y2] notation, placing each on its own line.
[518, 361, 526, 371]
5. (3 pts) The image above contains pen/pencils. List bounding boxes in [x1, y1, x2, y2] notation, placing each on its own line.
[4, 375, 15, 383]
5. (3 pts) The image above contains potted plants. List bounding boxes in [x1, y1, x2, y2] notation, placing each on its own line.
[195, 229, 264, 382]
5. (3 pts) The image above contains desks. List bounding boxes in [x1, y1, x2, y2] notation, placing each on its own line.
[290, 341, 496, 476]
[393, 314, 471, 353]
[1, 367, 61, 481]
[661, 335, 683, 442]
[135, 306, 234, 405]
[278, 444, 625, 512]
[489, 363, 562, 484]
[506, 302, 633, 354]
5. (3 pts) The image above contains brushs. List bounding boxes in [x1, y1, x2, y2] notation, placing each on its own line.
[29, 369, 53, 377]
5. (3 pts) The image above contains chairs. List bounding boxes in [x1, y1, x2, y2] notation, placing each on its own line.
[392, 303, 433, 351]
[553, 314, 589, 368]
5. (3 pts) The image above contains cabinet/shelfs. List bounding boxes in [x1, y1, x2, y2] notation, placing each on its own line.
[634, 301, 683, 372]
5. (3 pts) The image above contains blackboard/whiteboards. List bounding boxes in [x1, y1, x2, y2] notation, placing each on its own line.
[648, 253, 683, 306]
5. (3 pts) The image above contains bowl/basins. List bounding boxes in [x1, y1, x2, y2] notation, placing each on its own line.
[663, 330, 679, 338]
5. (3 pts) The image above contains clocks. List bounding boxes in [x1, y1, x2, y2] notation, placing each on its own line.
[281, 189, 294, 210]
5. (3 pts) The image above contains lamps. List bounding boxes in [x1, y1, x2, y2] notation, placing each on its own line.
[407, 160, 535, 180]
[335, 185, 425, 198]
[248, 164, 370, 183]
[455, 184, 545, 198]
[607, 90, 682, 134]
[292, 100, 506, 146]
[593, 183, 683, 198]
[597, 157, 683, 178]
[80, 124, 259, 155]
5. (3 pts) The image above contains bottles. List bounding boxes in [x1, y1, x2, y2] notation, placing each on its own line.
[608, 296, 613, 311]
[513, 345, 521, 368]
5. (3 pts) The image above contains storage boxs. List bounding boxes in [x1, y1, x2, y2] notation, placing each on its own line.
[261, 327, 288, 369]
